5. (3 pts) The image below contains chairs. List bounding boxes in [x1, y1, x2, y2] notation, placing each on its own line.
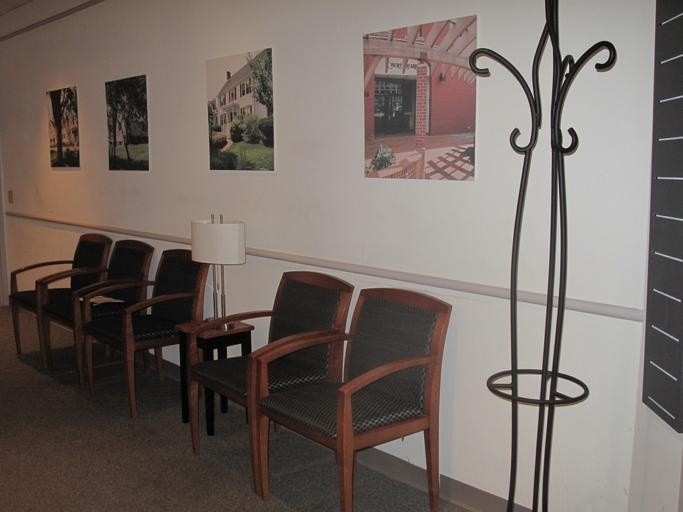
[256, 285, 455, 507]
[39, 236, 154, 390]
[12, 223, 112, 377]
[86, 247, 210, 416]
[185, 264, 354, 497]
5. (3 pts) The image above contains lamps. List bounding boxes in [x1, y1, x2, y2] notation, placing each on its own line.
[191, 218, 250, 334]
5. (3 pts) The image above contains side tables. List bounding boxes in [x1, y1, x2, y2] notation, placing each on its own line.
[173, 315, 254, 437]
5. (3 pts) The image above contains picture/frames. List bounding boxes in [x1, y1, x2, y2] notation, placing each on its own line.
[362, 15, 479, 182]
[102, 75, 151, 174]
[205, 46, 276, 174]
[47, 84, 82, 172]
